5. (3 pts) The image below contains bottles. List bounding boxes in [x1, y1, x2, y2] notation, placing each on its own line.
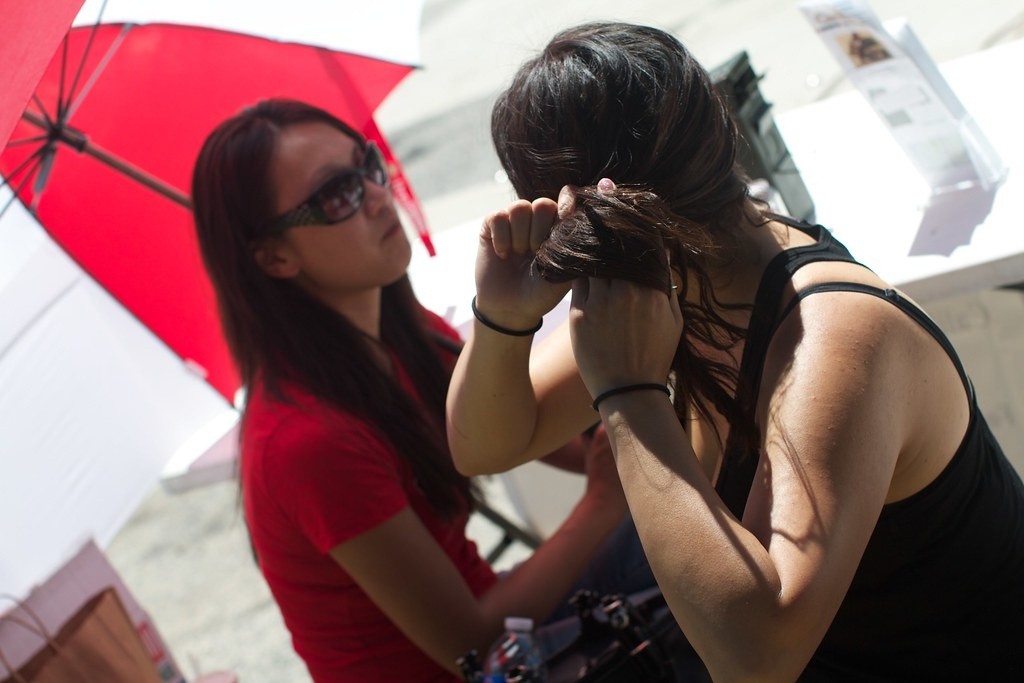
[484, 617, 548, 683]
[457, 649, 484, 683]
[749, 178, 792, 219]
[601, 594, 659, 658]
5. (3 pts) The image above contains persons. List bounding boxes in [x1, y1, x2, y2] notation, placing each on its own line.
[191, 98, 630, 683]
[445, 23, 1024, 683]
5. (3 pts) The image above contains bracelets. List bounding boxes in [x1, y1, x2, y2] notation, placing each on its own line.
[471, 295, 543, 336]
[592, 383, 671, 412]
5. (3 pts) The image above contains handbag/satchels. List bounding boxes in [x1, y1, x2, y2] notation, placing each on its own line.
[1, 592, 165, 683]
[457, 580, 712, 683]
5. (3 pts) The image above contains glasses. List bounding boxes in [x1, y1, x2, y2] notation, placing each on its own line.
[266, 142, 388, 237]
